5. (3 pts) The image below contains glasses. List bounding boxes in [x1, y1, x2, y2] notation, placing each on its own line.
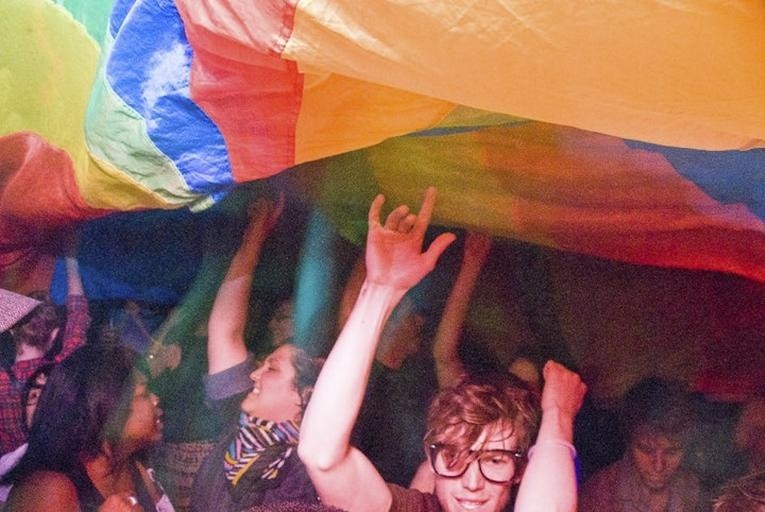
[428, 442, 521, 483]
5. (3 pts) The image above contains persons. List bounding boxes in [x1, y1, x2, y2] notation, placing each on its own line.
[337, 245, 429, 489]
[0, 364, 176, 512]
[0, 226, 91, 456]
[298, 185, 590, 512]
[266, 296, 293, 348]
[0, 367, 53, 511]
[576, 373, 714, 512]
[431, 233, 551, 396]
[186, 192, 323, 512]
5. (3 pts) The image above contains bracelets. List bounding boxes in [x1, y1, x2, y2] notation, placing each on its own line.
[527, 440, 577, 460]
